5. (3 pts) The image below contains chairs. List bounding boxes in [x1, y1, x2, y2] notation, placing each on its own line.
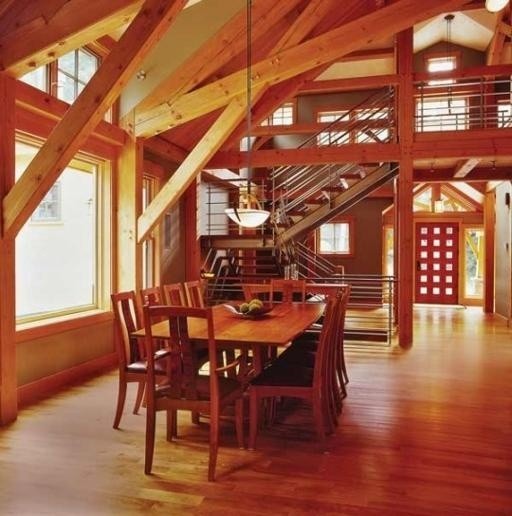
[110, 289, 174, 443]
[138, 280, 208, 326]
[140, 303, 245, 484]
[247, 294, 341, 456]
[268, 279, 351, 434]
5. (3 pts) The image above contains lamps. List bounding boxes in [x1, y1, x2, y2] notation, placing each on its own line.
[223, 0, 271, 230]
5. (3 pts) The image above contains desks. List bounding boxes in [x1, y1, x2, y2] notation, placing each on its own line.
[127, 301, 328, 451]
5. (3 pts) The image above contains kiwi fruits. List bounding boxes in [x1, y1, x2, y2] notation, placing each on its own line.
[240, 299, 263, 311]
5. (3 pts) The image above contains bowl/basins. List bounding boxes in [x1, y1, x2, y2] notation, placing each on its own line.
[222, 302, 277, 319]
